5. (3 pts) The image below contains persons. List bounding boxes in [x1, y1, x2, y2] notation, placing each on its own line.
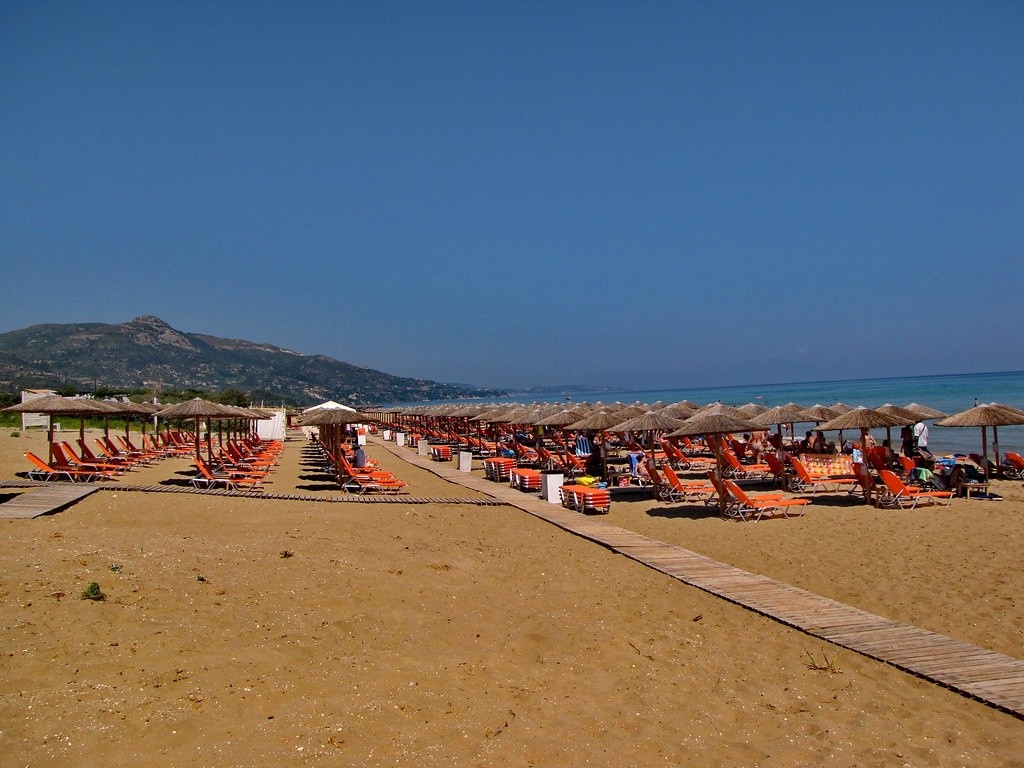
[974, 397, 980, 409]
[733, 404, 736, 409]
[834, 398, 837, 401]
[718, 400, 721, 403]
[393, 413, 963, 483]
[348, 444, 366, 468]
[765, 400, 768, 404]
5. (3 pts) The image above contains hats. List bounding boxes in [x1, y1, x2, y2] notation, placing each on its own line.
[351, 444, 360, 450]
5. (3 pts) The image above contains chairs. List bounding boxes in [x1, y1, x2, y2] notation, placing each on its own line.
[23, 415, 1024, 521]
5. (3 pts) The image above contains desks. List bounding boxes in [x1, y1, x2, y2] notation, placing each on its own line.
[957, 482, 993, 500]
[612, 473, 631, 488]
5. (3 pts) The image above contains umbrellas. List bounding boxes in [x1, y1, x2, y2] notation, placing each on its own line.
[0, 391, 380, 491]
[362, 400, 1024, 456]
